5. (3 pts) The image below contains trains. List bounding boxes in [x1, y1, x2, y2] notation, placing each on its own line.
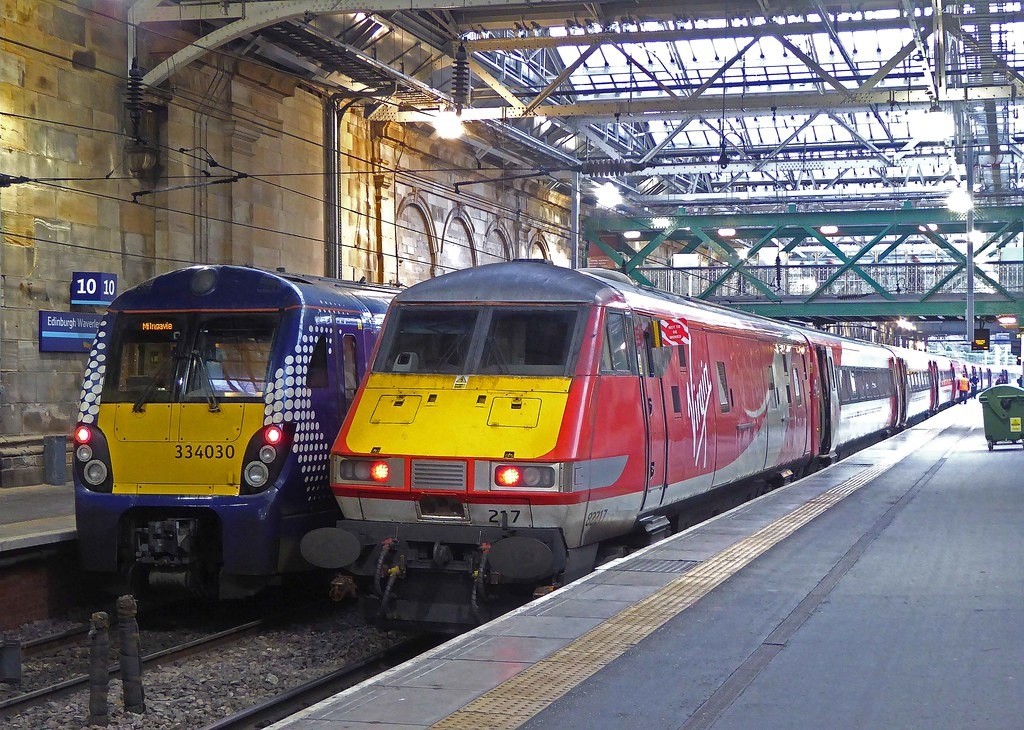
[69, 261, 406, 599]
[328, 256, 1022, 636]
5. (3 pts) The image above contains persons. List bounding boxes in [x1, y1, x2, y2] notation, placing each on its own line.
[1018, 377, 1022, 387]
[957, 374, 970, 404]
[969, 374, 979, 399]
[996, 375, 1007, 385]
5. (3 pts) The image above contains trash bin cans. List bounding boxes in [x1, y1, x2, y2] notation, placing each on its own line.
[979, 384, 1024, 452]
[43, 434, 67, 486]
